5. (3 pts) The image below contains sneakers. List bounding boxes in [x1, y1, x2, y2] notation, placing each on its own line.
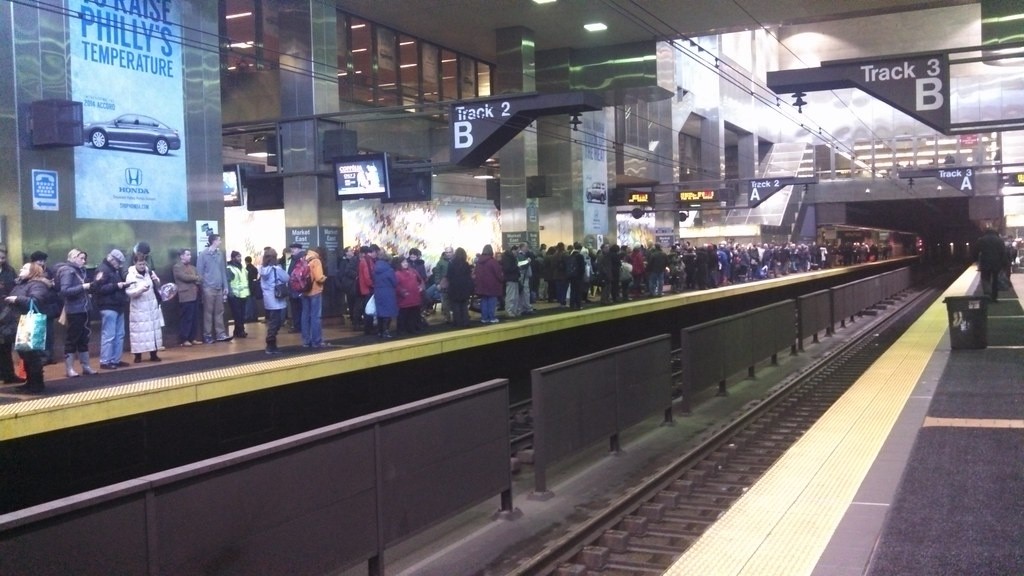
[481, 308, 538, 323]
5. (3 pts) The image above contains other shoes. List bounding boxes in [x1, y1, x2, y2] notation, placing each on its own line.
[99, 362, 117, 370]
[157, 331, 249, 351]
[150, 356, 162, 362]
[133, 356, 142, 363]
[265, 342, 330, 359]
[14, 379, 46, 394]
[568, 291, 666, 313]
[117, 361, 130, 367]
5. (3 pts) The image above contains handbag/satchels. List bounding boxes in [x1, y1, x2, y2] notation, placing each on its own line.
[149, 269, 163, 305]
[618, 264, 633, 284]
[274, 283, 290, 297]
[364, 294, 377, 316]
[13, 298, 47, 351]
[518, 282, 524, 293]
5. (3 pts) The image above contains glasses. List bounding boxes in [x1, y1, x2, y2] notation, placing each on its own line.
[445, 252, 453, 253]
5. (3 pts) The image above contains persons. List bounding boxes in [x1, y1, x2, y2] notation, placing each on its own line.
[336, 241, 537, 339]
[196, 234, 235, 342]
[732, 240, 894, 285]
[972, 227, 1019, 300]
[225, 244, 331, 354]
[0, 241, 166, 394]
[534, 241, 739, 311]
[174, 248, 205, 347]
[944, 153, 955, 164]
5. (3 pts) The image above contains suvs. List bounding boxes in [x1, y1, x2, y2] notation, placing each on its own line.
[586, 180, 607, 204]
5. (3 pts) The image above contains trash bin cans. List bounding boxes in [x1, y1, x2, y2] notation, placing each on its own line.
[942, 296, 991, 348]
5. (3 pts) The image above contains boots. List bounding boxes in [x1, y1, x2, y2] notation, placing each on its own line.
[64, 352, 80, 377]
[79, 351, 98, 375]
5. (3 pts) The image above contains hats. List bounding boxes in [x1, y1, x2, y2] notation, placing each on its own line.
[231, 251, 240, 257]
[264, 247, 278, 256]
[30, 250, 48, 260]
[390, 254, 405, 265]
[111, 249, 126, 262]
[707, 244, 714, 251]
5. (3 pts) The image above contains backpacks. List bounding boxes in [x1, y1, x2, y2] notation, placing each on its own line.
[289, 256, 321, 292]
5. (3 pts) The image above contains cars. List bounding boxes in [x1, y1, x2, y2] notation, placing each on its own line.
[83, 113, 181, 157]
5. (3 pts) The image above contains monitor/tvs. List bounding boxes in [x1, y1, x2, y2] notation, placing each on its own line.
[333, 152, 390, 201]
[223, 164, 244, 206]
[381, 158, 433, 203]
